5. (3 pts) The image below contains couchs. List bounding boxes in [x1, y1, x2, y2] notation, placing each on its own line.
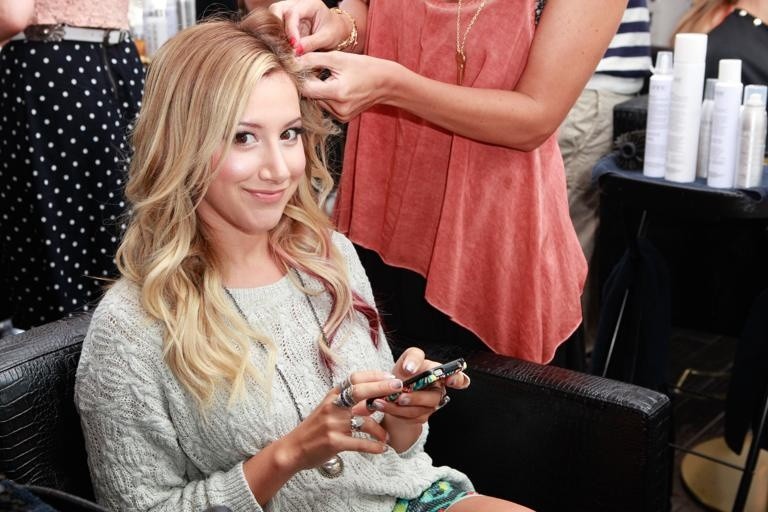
[0, 292, 680, 510]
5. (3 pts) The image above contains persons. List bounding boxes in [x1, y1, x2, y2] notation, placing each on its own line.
[669, 0, 768, 88]
[535, 2, 656, 368]
[74, 7, 536, 511]
[250, 2, 629, 398]
[2, 0, 153, 328]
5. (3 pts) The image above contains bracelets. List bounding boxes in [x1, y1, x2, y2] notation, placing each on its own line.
[330, 3, 361, 52]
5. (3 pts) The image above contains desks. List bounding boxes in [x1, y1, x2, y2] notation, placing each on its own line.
[586, 150, 767, 511]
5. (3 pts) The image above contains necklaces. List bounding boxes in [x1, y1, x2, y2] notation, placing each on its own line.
[450, 0, 487, 81]
[214, 264, 344, 479]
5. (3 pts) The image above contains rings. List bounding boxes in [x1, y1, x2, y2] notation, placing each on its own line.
[333, 385, 358, 410]
[438, 389, 451, 409]
[339, 374, 352, 390]
[457, 371, 471, 389]
[349, 414, 364, 431]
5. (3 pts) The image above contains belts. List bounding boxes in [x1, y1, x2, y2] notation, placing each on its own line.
[11, 25, 121, 46]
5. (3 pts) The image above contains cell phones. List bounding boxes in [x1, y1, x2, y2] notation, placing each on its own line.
[364, 357, 468, 412]
